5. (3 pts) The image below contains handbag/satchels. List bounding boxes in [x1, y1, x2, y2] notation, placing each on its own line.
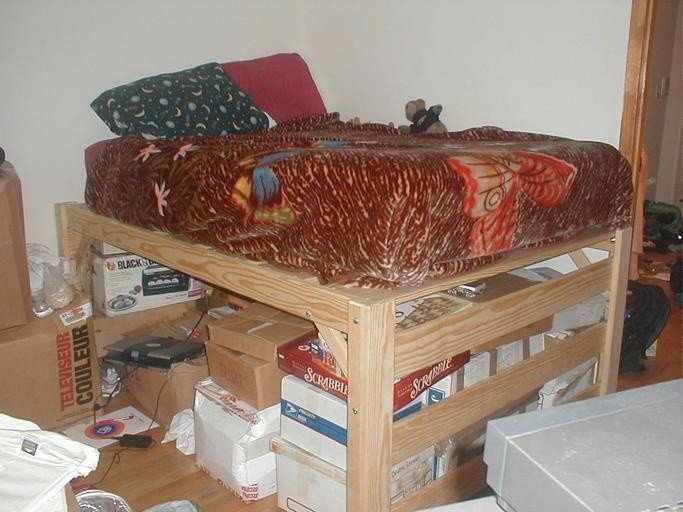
[618, 278, 672, 373]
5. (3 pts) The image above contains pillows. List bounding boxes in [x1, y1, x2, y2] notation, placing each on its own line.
[89, 62, 278, 140]
[220, 52, 329, 125]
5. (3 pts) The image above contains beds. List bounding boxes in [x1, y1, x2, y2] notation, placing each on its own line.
[53, 115, 634, 512]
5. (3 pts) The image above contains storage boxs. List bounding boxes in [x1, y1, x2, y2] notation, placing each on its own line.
[396, 267, 612, 477]
[204, 338, 282, 414]
[112, 361, 208, 428]
[193, 376, 281, 505]
[208, 299, 319, 364]
[1, 161, 34, 329]
[276, 375, 423, 472]
[2, 283, 105, 435]
[80, 230, 204, 320]
[269, 436, 437, 511]
[276, 330, 472, 416]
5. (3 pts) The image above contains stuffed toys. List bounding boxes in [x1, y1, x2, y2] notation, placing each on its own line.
[397, 97, 447, 135]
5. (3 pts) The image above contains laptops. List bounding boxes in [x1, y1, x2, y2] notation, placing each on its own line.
[104, 333, 203, 371]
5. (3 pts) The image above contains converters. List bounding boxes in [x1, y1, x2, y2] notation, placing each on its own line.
[119, 431, 152, 449]
